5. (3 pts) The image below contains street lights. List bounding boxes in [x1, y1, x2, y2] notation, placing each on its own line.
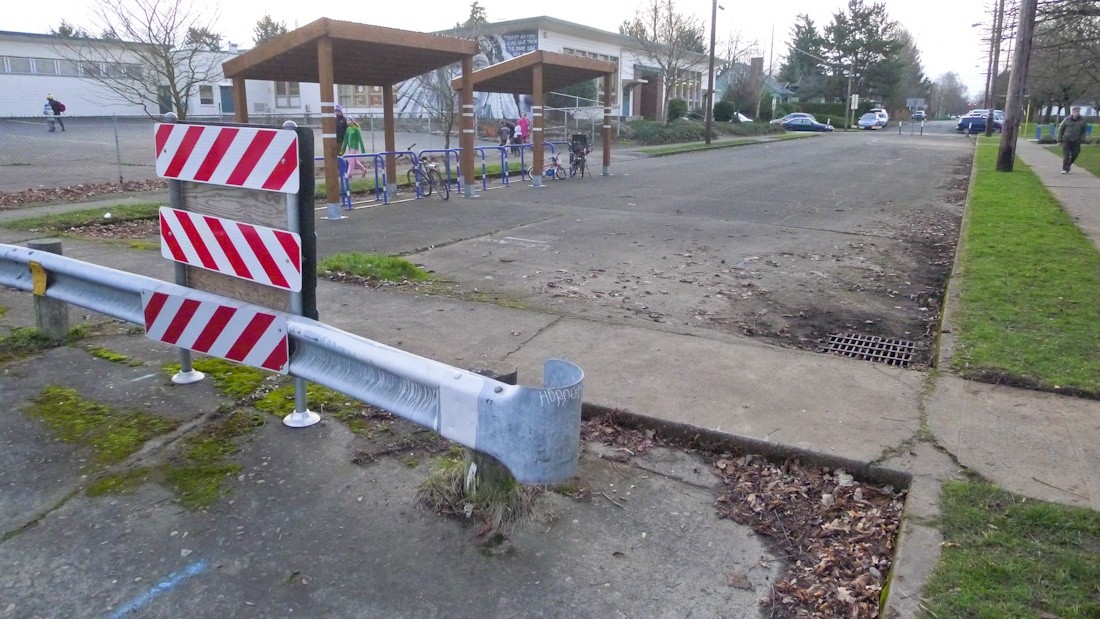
[706, 5, 724, 144]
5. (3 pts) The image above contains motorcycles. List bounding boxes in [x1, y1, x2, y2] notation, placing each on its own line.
[567, 134, 592, 178]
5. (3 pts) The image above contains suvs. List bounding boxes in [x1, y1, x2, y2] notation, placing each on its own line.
[870, 108, 888, 127]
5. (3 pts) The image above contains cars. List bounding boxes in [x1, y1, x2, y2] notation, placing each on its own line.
[730, 112, 753, 122]
[857, 113, 880, 129]
[912, 110, 927, 120]
[770, 112, 834, 131]
[956, 109, 1021, 135]
[951, 114, 961, 120]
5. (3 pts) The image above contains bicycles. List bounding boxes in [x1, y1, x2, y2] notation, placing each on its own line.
[395, 143, 449, 200]
[527, 152, 566, 180]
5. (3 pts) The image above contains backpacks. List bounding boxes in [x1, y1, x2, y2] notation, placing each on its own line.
[56, 102, 65, 112]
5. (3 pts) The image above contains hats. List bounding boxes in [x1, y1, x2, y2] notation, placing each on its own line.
[47, 93, 53, 100]
[335, 105, 342, 112]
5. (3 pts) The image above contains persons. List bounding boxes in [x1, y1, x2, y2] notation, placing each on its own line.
[47, 95, 64, 131]
[43, 104, 55, 132]
[497, 112, 530, 157]
[339, 116, 367, 179]
[335, 105, 347, 156]
[1057, 107, 1088, 174]
